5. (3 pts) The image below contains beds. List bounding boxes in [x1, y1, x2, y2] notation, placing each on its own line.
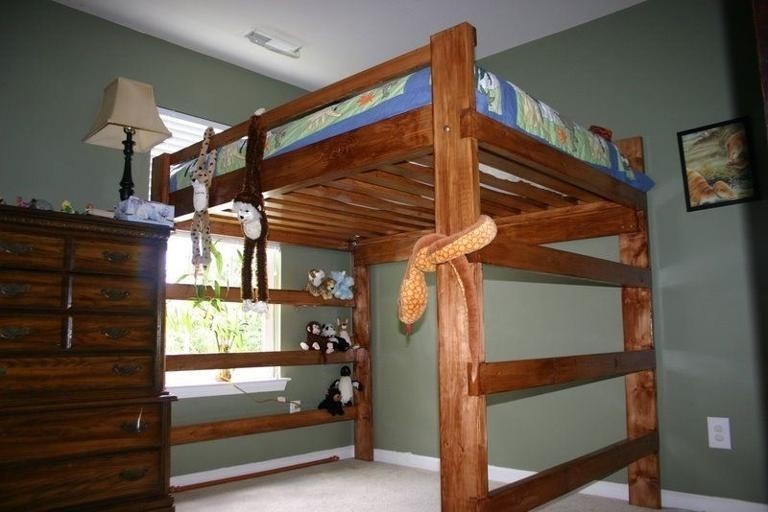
[151, 17, 664, 511]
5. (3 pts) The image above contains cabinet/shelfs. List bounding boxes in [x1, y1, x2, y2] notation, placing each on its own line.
[1, 394, 174, 506]
[2, 216, 159, 396]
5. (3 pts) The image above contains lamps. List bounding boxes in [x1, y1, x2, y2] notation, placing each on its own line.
[80, 76, 172, 219]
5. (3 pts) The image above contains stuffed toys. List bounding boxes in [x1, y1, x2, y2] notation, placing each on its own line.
[300, 269, 366, 417]
[235, 108, 268, 315]
[396, 211, 498, 332]
[187, 128, 218, 271]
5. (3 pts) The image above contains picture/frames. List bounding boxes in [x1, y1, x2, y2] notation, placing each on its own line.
[677, 114, 768, 212]
